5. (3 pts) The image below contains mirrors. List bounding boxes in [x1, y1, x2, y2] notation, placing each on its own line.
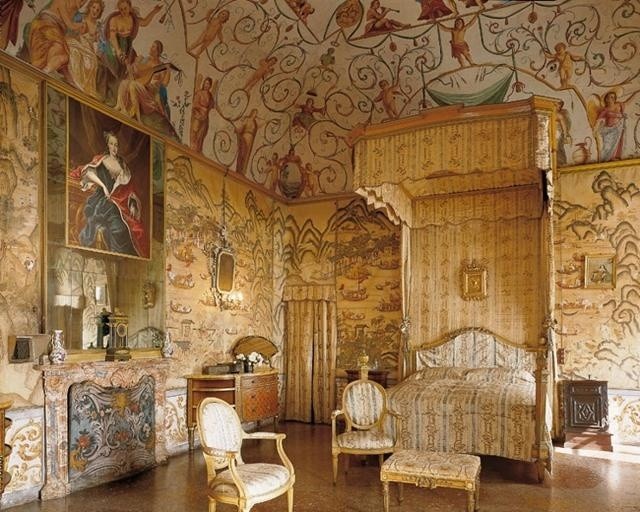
[39, 80, 171, 360]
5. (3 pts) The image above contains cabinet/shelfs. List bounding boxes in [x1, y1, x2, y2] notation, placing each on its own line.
[188, 376, 280, 431]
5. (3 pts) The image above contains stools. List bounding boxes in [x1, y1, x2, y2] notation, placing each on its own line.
[378, 447, 484, 512]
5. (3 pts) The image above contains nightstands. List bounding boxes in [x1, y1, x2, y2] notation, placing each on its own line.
[562, 380, 613, 452]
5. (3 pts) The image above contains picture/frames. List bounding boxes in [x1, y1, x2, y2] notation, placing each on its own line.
[583, 252, 618, 291]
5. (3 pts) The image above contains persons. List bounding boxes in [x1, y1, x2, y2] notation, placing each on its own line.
[78, 128, 148, 256]
[591, 265, 608, 284]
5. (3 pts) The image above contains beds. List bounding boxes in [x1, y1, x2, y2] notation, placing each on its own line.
[379, 326, 555, 487]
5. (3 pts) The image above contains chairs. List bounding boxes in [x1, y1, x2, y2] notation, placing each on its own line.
[329, 376, 408, 504]
[196, 396, 297, 512]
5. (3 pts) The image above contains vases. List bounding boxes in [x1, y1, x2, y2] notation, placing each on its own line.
[48, 330, 65, 364]
[161, 332, 173, 358]
